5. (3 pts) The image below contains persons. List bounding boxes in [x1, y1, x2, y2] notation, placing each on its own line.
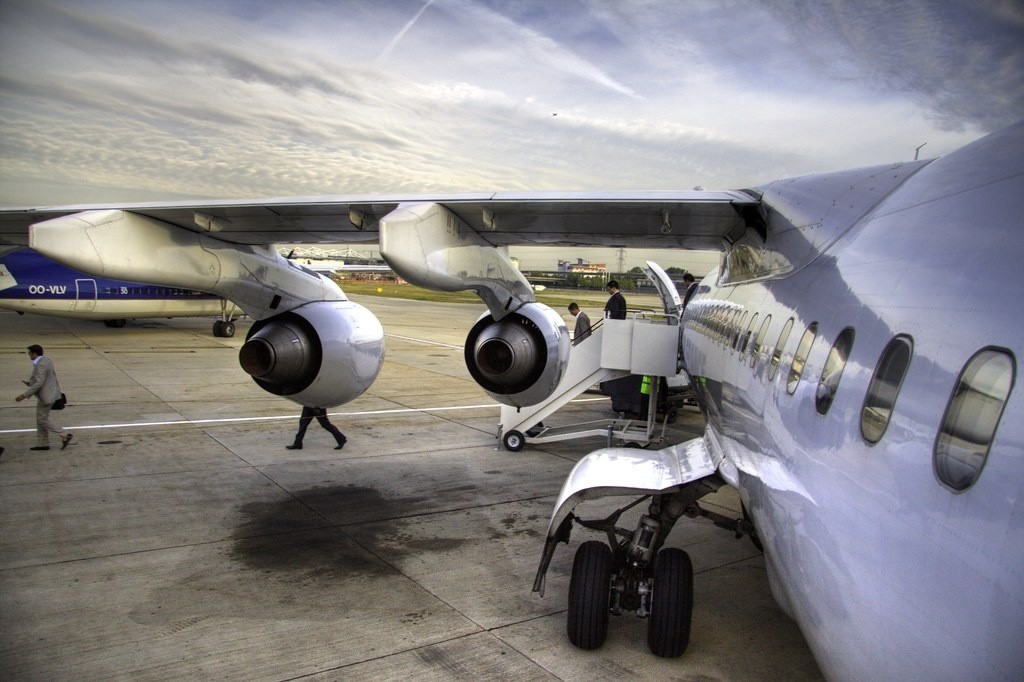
[286, 406, 347, 449]
[605, 281, 626, 320]
[639, 376, 660, 428]
[683, 274, 697, 310]
[15, 345, 72, 451]
[568, 303, 590, 345]
[689, 286, 710, 300]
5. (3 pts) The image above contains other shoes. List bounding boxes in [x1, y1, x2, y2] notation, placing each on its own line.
[30, 446, 49, 450]
[335, 440, 346, 449]
[61, 434, 73, 449]
[286, 444, 302, 449]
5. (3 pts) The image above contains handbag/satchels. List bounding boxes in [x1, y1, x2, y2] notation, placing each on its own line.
[51, 393, 66, 410]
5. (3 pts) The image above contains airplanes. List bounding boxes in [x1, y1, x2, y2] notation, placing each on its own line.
[0, 246, 392, 338]
[0, 117, 1024, 682]
